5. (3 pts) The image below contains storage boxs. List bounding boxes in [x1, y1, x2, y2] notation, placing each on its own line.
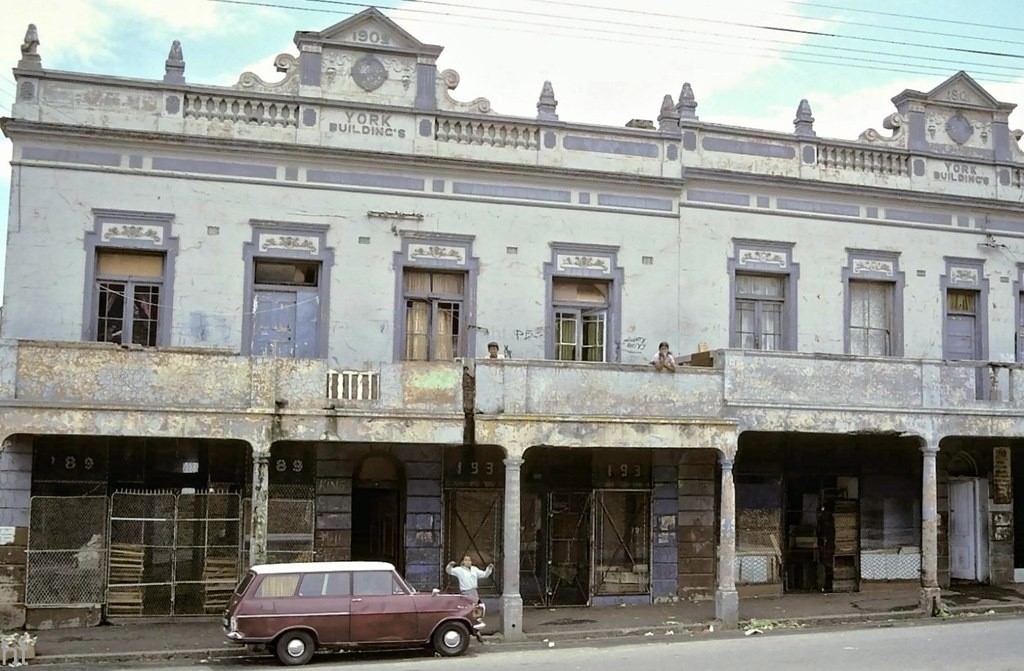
[795, 537, 818, 548]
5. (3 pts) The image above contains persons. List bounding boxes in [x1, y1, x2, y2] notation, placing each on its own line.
[484, 341, 504, 359]
[446, 555, 494, 603]
[650, 342, 677, 373]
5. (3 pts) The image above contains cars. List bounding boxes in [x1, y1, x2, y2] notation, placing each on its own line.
[222, 562, 486, 666]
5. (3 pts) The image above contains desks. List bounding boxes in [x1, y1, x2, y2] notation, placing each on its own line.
[785, 550, 821, 591]
